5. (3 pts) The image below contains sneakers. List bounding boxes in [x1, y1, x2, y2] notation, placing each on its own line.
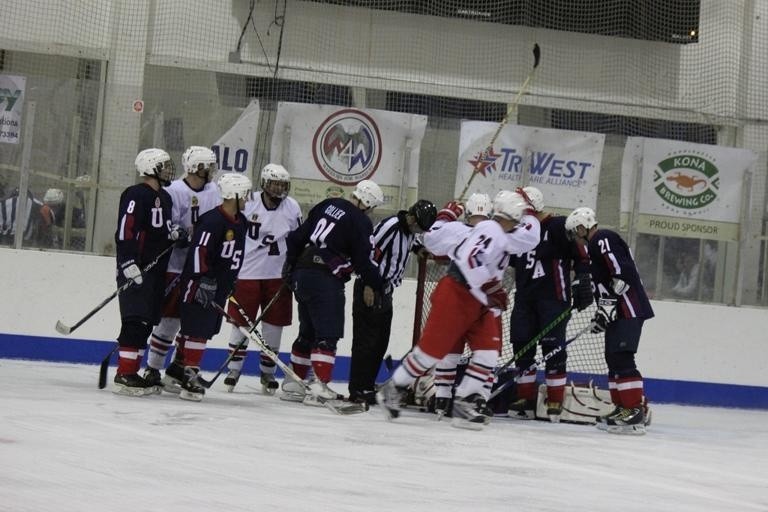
[224, 369, 246, 387]
[305, 376, 344, 399]
[260, 369, 278, 389]
[381, 382, 401, 419]
[164, 360, 185, 383]
[142, 365, 165, 387]
[433, 395, 448, 410]
[451, 397, 486, 421]
[508, 397, 534, 411]
[605, 406, 645, 424]
[114, 370, 158, 389]
[180, 371, 208, 396]
[593, 405, 622, 425]
[280, 375, 307, 393]
[545, 400, 564, 413]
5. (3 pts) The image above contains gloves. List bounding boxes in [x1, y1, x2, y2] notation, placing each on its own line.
[607, 276, 634, 300]
[359, 272, 390, 314]
[569, 272, 595, 313]
[477, 279, 510, 313]
[193, 275, 218, 311]
[589, 297, 619, 335]
[434, 199, 466, 224]
[134, 261, 144, 286]
[515, 184, 539, 218]
[272, 278, 294, 304]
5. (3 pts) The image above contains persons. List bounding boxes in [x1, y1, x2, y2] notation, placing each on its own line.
[378, 190, 527, 430]
[565, 207, 656, 438]
[701, 240, 718, 286]
[348, 198, 438, 402]
[283, 180, 383, 406]
[671, 254, 713, 302]
[112, 147, 174, 393]
[224, 164, 303, 393]
[1, 186, 34, 245]
[44, 187, 83, 227]
[511, 186, 593, 415]
[144, 171, 253, 402]
[424, 192, 495, 420]
[145, 145, 217, 388]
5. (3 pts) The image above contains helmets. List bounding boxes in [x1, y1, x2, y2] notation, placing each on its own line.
[12, 186, 34, 199]
[75, 175, 91, 189]
[564, 206, 600, 230]
[464, 191, 495, 219]
[492, 189, 535, 224]
[132, 147, 177, 183]
[521, 185, 545, 213]
[216, 172, 254, 202]
[260, 162, 293, 202]
[180, 144, 218, 181]
[413, 198, 439, 233]
[42, 189, 65, 207]
[351, 178, 385, 210]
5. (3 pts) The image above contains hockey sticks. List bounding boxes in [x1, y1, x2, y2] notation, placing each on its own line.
[196, 288, 281, 389]
[55, 242, 173, 335]
[456, 41, 540, 200]
[211, 301, 365, 414]
[99, 272, 182, 389]
